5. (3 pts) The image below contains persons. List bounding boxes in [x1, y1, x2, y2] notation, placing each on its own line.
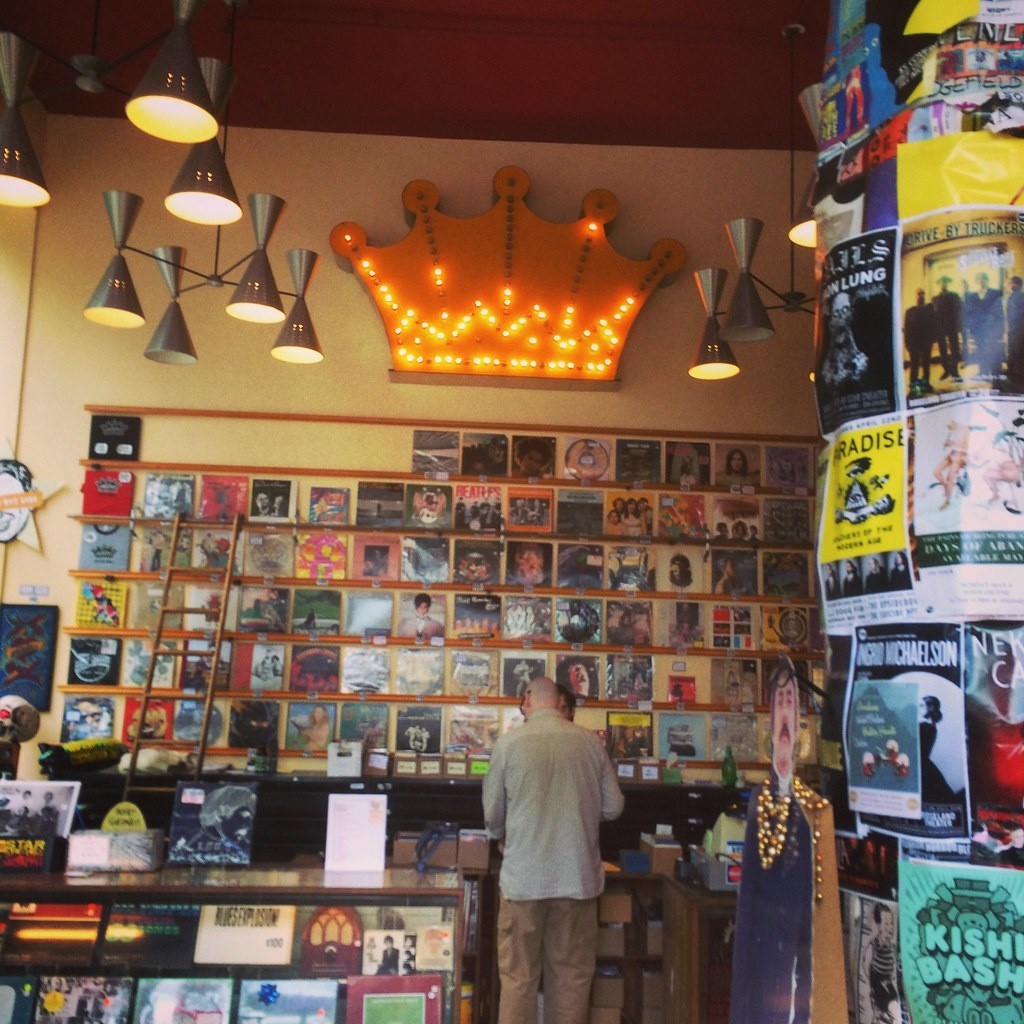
[255, 654, 283, 682]
[715, 555, 739, 595]
[715, 522, 760, 545]
[396, 593, 445, 638]
[726, 653, 849, 1023]
[905, 275, 1024, 396]
[667, 554, 694, 593]
[149, 530, 165, 570]
[932, 419, 1019, 510]
[557, 655, 597, 697]
[481, 676, 624, 1024]
[37, 976, 130, 1024]
[511, 438, 551, 479]
[817, 293, 893, 402]
[454, 496, 502, 528]
[376, 937, 399, 977]
[0, 790, 59, 836]
[716, 447, 757, 486]
[300, 705, 330, 751]
[608, 496, 655, 536]
[823, 549, 911, 601]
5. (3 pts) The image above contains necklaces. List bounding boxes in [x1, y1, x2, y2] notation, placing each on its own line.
[753, 777, 831, 905]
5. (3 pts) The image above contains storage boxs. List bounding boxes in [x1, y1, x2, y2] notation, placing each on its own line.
[1, 835, 68, 873]
[394, 829, 683, 1024]
[68, 830, 164, 871]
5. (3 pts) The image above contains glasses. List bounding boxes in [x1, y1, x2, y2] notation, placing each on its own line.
[518, 690, 531, 715]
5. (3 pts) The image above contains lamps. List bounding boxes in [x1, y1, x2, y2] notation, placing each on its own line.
[1, 0, 324, 364]
[647, 22, 816, 380]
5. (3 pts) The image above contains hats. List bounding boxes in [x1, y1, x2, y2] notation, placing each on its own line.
[768, 656, 796, 701]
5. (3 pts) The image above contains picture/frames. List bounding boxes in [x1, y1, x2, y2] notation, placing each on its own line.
[0, 779, 83, 838]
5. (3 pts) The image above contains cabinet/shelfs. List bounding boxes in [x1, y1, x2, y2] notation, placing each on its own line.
[60, 397, 828, 848]
[0, 862, 496, 1024]
